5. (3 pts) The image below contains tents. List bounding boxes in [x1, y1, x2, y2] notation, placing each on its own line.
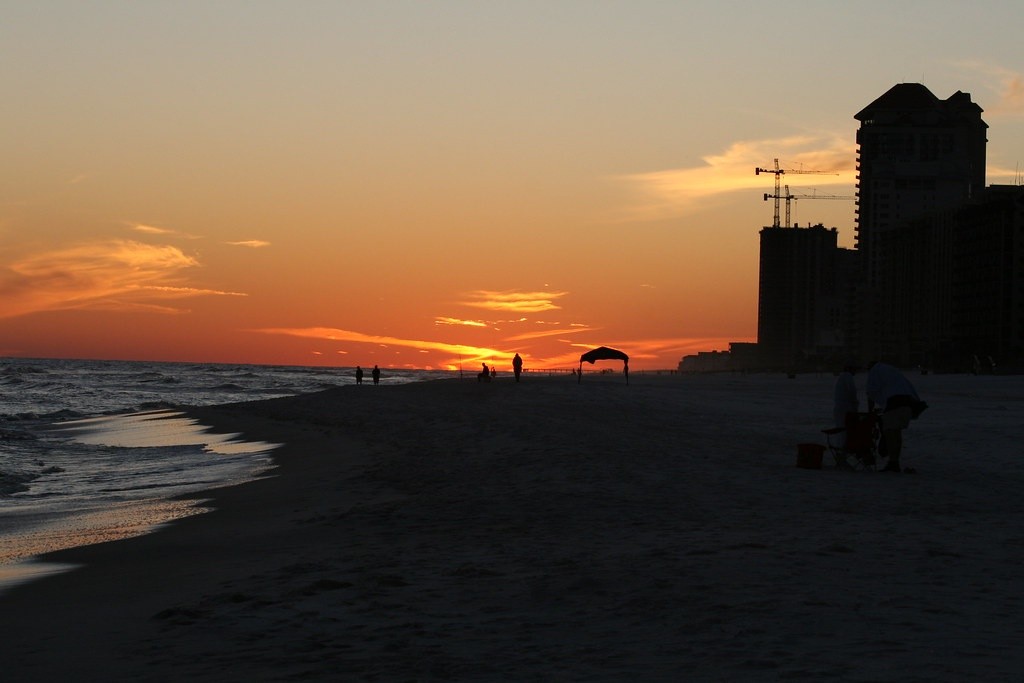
[579, 347, 630, 387]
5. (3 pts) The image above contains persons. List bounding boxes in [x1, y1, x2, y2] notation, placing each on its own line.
[355, 365, 363, 383]
[987, 355, 1000, 375]
[372, 365, 381, 386]
[513, 353, 522, 383]
[830, 356, 926, 476]
[477, 364, 489, 383]
